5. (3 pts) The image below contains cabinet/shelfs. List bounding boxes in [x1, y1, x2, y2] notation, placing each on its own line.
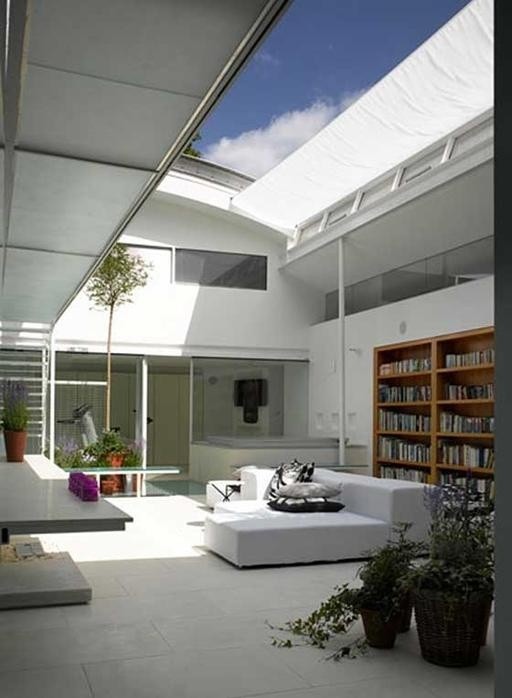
[372, 326, 495, 486]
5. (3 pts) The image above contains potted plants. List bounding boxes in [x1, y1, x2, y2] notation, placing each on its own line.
[86, 429, 141, 494]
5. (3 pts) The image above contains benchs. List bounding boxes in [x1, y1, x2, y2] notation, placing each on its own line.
[205, 498, 387, 569]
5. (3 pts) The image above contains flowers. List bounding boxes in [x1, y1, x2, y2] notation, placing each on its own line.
[0, 378, 32, 432]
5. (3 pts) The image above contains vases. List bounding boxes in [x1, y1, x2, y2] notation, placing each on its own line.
[4, 430, 27, 462]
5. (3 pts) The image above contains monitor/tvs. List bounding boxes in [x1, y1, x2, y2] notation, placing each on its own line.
[234, 379, 265, 406]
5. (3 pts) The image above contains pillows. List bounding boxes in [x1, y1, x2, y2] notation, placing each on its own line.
[265, 459, 315, 499]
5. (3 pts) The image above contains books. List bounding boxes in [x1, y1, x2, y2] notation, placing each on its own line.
[377, 349, 496, 523]
